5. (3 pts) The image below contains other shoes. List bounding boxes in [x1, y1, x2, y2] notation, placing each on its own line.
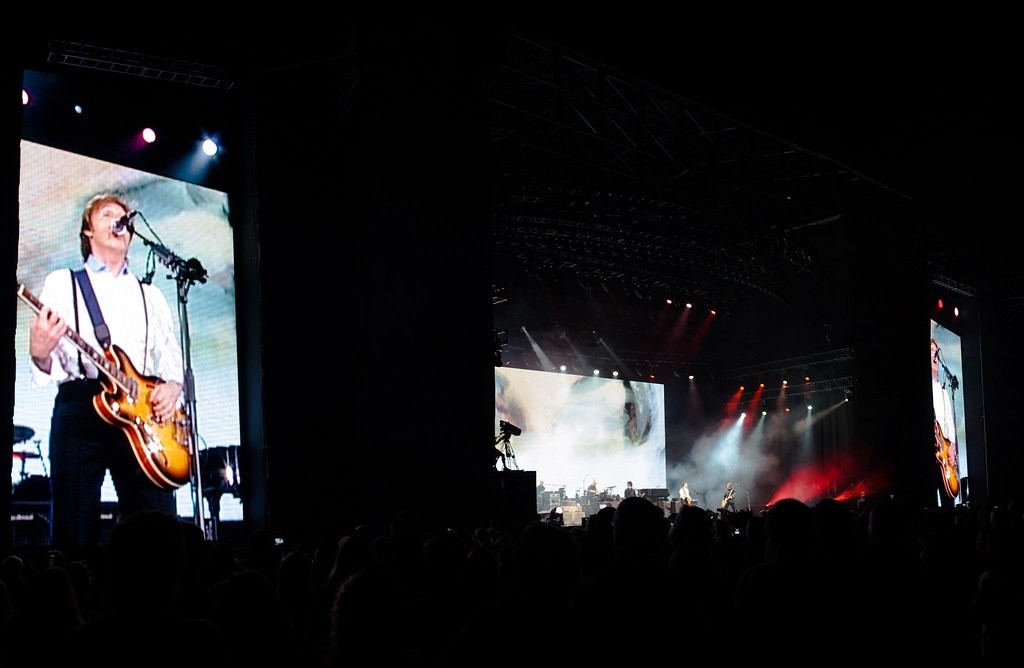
[503, 468, 511, 470]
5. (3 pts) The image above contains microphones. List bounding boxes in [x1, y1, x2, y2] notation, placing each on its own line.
[933, 348, 940, 363]
[111, 210, 137, 236]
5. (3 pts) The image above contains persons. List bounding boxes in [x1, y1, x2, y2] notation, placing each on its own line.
[930, 340, 963, 516]
[0, 496, 1024, 668]
[624, 481, 637, 499]
[679, 482, 692, 507]
[587, 479, 597, 497]
[722, 482, 738, 512]
[30, 190, 185, 527]
[535, 479, 545, 495]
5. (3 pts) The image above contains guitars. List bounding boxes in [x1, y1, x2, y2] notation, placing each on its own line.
[14, 283, 193, 489]
[934, 415, 961, 499]
[680, 485, 691, 505]
[721, 493, 736, 509]
